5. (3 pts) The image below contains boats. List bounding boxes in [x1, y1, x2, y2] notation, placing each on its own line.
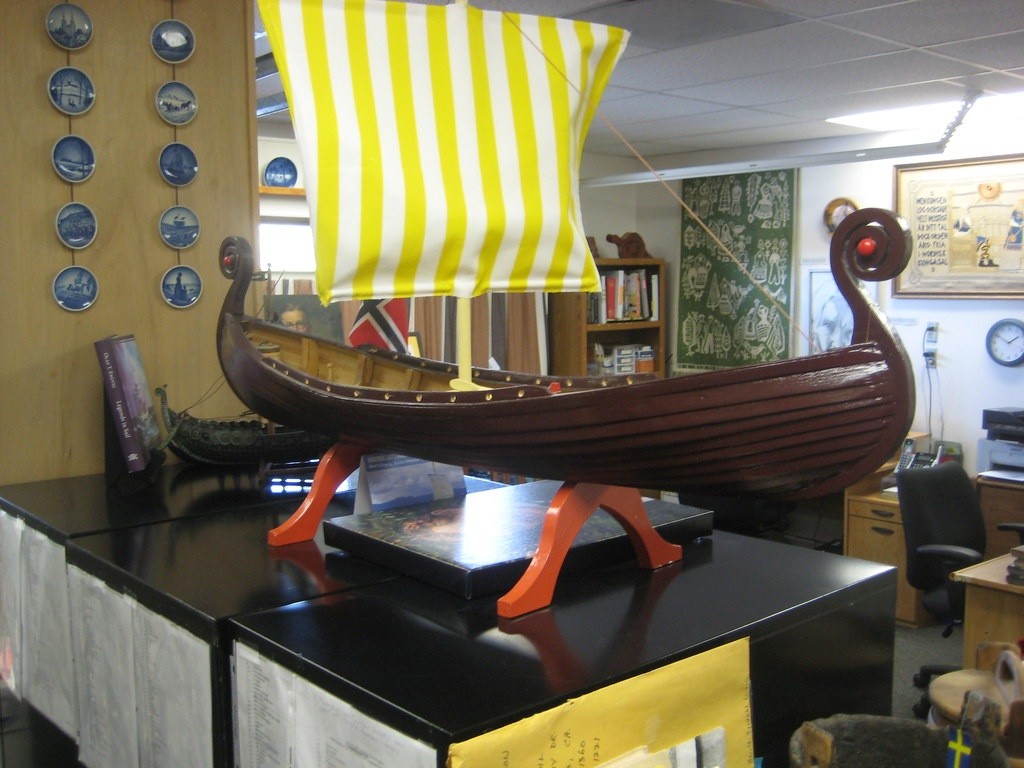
[214, 207, 917, 513]
[151, 386, 332, 471]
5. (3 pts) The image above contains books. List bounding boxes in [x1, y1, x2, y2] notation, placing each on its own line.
[588, 269, 659, 324]
[95, 332, 165, 477]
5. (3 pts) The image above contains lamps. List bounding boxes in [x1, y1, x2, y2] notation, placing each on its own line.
[579, 91, 981, 190]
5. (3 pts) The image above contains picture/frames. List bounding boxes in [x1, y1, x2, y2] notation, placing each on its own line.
[807, 269, 879, 354]
[892, 152, 1024, 300]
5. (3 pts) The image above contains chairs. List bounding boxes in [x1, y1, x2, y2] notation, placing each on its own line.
[897, 459, 985, 717]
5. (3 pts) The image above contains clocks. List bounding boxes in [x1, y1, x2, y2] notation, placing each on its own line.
[986, 319, 1024, 366]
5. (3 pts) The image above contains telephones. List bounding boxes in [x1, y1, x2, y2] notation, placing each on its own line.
[893, 438, 943, 473]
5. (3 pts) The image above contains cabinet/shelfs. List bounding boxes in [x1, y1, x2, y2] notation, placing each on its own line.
[978, 477, 1024, 563]
[547, 258, 667, 379]
[950, 551, 1023, 673]
[843, 432, 937, 631]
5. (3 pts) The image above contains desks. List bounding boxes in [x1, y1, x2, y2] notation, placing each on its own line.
[0, 452, 899, 768]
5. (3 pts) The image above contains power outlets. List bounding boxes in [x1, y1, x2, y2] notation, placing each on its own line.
[926, 322, 940, 342]
